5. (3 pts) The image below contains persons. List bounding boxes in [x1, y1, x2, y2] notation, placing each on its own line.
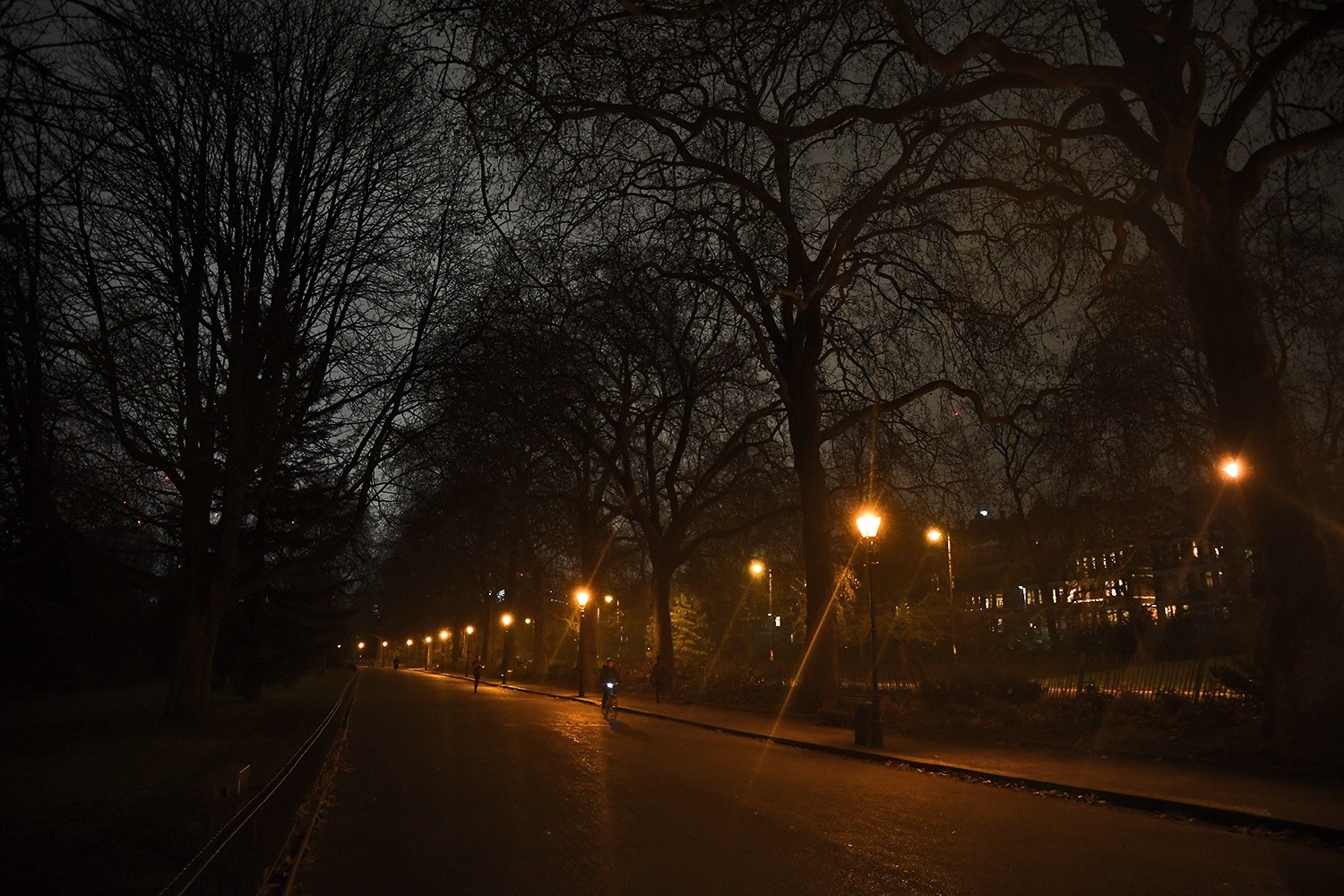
[600, 658, 618, 710]
[650, 654, 660, 703]
[472, 653, 485, 693]
[394, 655, 399, 673]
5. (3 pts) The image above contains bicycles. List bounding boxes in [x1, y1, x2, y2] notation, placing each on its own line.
[598, 679, 624, 721]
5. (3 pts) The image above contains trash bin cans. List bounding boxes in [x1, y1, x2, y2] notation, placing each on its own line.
[852, 701, 877, 748]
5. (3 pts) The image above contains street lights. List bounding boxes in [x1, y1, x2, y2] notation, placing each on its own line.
[524, 618, 537, 676]
[923, 528, 957, 665]
[439, 630, 450, 674]
[464, 624, 475, 676]
[576, 592, 590, 699]
[1219, 452, 1263, 600]
[406, 639, 413, 669]
[603, 594, 622, 669]
[358, 641, 366, 665]
[854, 508, 886, 746]
[501, 614, 513, 686]
[382, 641, 387, 666]
[749, 561, 775, 681]
[424, 636, 432, 670]
